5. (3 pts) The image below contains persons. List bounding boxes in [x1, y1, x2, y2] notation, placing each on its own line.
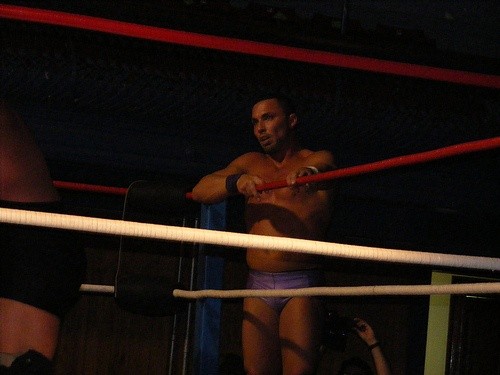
[320, 316, 390, 375]
[0, 100, 86, 375]
[192, 93, 339, 375]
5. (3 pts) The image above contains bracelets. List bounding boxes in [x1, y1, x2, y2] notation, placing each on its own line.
[225, 174, 241, 194]
[367, 342, 380, 351]
[305, 165, 319, 175]
